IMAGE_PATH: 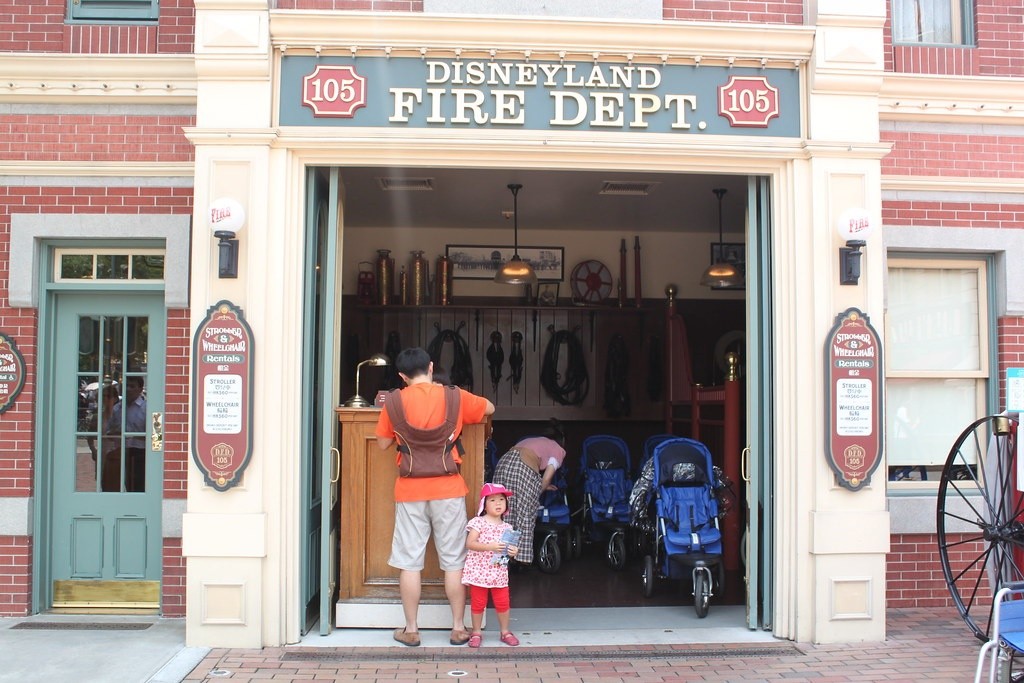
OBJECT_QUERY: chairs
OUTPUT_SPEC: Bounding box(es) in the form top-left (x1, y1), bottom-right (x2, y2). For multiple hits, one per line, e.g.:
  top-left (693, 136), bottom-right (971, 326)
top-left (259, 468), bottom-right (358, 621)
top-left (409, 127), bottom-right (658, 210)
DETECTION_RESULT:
top-left (974, 580), bottom-right (1024, 683)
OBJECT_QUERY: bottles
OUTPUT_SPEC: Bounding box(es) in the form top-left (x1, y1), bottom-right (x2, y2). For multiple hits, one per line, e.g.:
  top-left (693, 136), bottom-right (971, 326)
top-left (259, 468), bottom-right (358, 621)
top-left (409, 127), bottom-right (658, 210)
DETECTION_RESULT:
top-left (377, 248), bottom-right (452, 306)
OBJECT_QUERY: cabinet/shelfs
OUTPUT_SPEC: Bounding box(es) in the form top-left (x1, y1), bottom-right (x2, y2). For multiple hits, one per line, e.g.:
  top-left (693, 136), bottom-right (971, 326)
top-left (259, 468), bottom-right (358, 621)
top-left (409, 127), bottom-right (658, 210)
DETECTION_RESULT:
top-left (335, 405), bottom-right (492, 631)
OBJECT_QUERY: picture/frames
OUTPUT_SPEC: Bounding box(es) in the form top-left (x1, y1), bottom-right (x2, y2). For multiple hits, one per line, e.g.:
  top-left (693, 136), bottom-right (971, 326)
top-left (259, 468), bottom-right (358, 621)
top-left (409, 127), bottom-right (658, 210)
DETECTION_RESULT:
top-left (537, 283), bottom-right (559, 305)
top-left (710, 243), bottom-right (745, 290)
top-left (445, 244), bottom-right (564, 282)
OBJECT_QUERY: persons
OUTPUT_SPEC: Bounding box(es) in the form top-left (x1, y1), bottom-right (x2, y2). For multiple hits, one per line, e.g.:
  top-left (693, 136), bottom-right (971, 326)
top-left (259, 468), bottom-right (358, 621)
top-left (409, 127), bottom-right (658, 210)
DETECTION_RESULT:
top-left (459, 483), bottom-right (520, 648)
top-left (373, 348), bottom-right (496, 647)
top-left (491, 416), bottom-right (569, 575)
top-left (87, 373), bottom-right (147, 493)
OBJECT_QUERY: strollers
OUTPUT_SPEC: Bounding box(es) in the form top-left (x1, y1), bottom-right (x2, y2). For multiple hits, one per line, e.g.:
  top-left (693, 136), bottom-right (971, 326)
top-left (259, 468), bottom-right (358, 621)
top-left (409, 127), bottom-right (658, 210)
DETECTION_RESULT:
top-left (631, 438), bottom-right (741, 619)
top-left (485, 433), bottom-right (685, 573)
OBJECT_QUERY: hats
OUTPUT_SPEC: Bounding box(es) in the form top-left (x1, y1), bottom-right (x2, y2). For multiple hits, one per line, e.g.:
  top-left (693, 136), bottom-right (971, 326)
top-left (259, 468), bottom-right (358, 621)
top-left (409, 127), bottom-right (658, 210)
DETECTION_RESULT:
top-left (477, 483), bottom-right (513, 516)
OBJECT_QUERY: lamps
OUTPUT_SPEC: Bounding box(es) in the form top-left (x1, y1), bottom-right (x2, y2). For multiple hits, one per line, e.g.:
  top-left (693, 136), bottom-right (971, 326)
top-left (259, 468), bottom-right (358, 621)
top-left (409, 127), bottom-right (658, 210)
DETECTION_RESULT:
top-left (207, 199), bottom-right (245, 278)
top-left (494, 185), bottom-right (539, 283)
top-left (345, 353), bottom-right (392, 406)
top-left (699, 188), bottom-right (745, 287)
top-left (838, 204), bottom-right (876, 284)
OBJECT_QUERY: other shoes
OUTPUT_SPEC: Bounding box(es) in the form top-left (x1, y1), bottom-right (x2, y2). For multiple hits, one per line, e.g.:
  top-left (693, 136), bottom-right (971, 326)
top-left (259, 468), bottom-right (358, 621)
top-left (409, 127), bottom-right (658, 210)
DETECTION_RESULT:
top-left (394, 626), bottom-right (420, 646)
top-left (468, 633), bottom-right (481, 647)
top-left (500, 632), bottom-right (518, 646)
top-left (450, 626), bottom-right (473, 645)
